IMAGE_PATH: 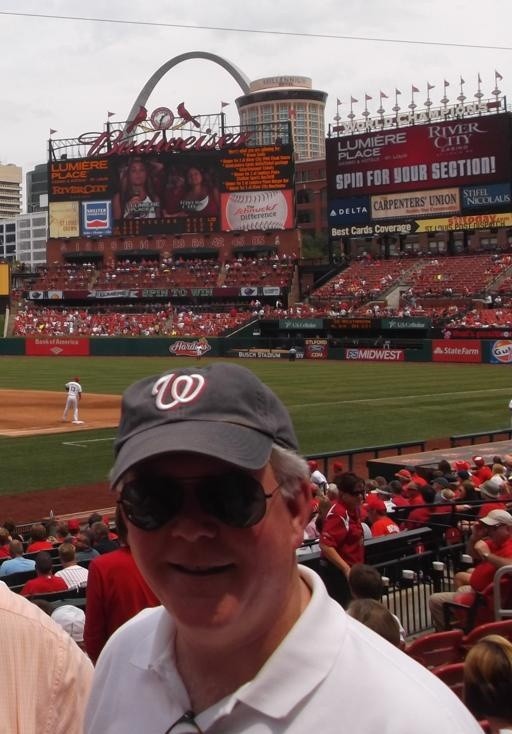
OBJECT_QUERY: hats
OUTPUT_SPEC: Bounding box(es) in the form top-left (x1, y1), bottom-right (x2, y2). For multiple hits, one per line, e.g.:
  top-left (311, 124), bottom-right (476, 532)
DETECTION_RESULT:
top-left (451, 461), bottom-right (472, 471)
top-left (441, 489), bottom-right (456, 501)
top-left (50, 604), bottom-right (86, 642)
top-left (333, 463), bottom-right (342, 472)
top-left (109, 361), bottom-right (300, 494)
top-left (478, 482), bottom-right (500, 498)
top-left (489, 474), bottom-right (508, 489)
top-left (395, 469), bottom-right (411, 480)
top-left (309, 460), bottom-right (317, 470)
top-left (68, 518), bottom-right (79, 530)
top-left (472, 456), bottom-right (485, 467)
top-left (430, 477), bottom-right (448, 488)
top-left (479, 510), bottom-right (512, 526)
top-left (363, 495), bottom-right (385, 510)
top-left (406, 481), bottom-right (419, 490)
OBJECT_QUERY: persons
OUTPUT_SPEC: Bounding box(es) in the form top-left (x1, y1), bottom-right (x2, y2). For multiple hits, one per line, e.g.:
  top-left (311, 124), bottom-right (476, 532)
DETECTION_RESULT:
top-left (196, 345), bottom-right (202, 361)
top-left (113, 156), bottom-right (222, 217)
top-left (289, 347), bottom-right (296, 361)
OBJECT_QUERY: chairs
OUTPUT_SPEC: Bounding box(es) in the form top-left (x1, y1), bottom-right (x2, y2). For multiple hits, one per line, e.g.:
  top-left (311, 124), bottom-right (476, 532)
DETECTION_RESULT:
top-left (1, 524), bottom-right (128, 615)
top-left (398, 620), bottom-right (512, 734)
top-left (443, 578), bottom-right (512, 634)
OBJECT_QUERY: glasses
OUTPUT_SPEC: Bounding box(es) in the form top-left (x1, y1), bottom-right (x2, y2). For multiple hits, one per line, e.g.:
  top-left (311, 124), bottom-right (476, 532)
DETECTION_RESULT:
top-left (114, 471), bottom-right (293, 531)
top-left (166, 711), bottom-right (203, 734)
